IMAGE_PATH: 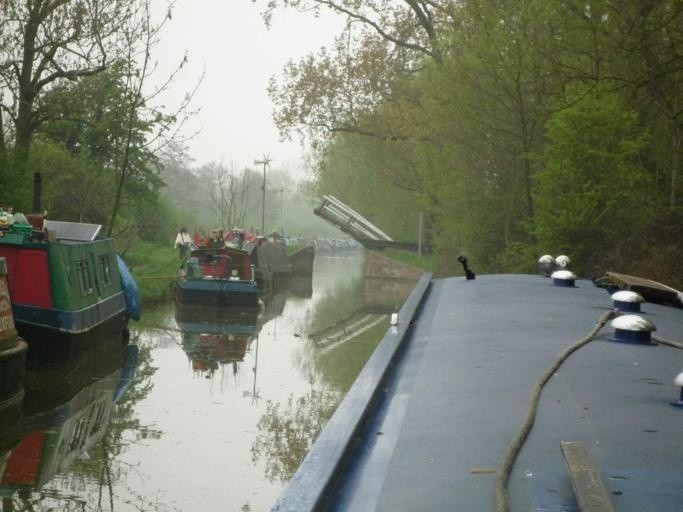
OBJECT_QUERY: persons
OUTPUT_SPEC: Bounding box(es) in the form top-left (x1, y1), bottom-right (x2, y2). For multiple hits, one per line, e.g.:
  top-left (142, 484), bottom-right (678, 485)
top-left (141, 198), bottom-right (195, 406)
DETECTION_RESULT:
top-left (172, 225), bottom-right (193, 269)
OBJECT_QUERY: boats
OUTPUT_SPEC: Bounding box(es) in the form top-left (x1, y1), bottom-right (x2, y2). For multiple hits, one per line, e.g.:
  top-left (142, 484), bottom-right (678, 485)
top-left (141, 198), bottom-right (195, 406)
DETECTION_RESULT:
top-left (0, 172), bottom-right (315, 407)
